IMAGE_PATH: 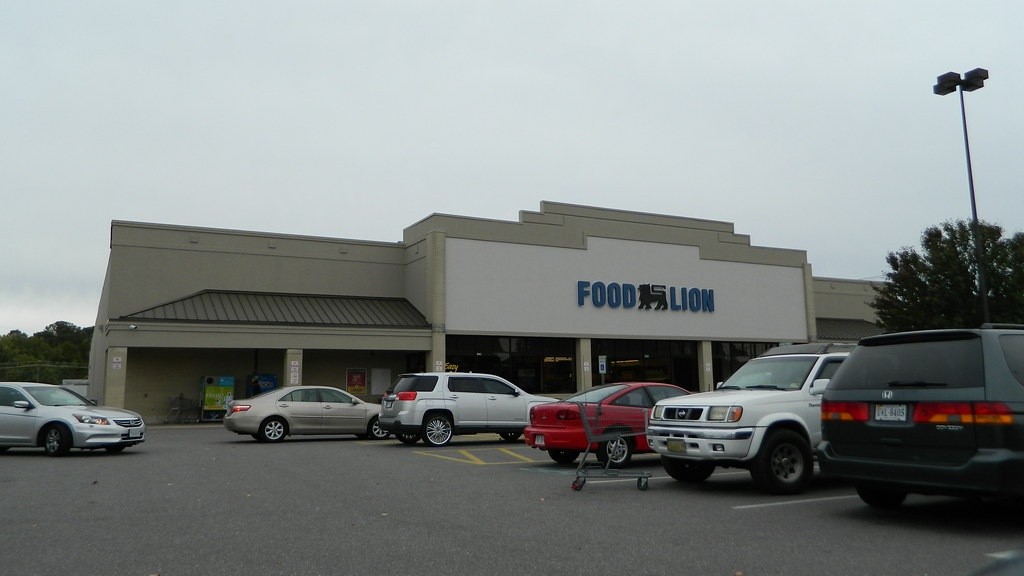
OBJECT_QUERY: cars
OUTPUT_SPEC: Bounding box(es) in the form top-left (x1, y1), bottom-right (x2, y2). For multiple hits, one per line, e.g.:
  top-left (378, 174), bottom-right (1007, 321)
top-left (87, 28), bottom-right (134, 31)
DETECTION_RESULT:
top-left (523, 379), bottom-right (696, 471)
top-left (0, 379), bottom-right (146, 459)
top-left (222, 383), bottom-right (391, 444)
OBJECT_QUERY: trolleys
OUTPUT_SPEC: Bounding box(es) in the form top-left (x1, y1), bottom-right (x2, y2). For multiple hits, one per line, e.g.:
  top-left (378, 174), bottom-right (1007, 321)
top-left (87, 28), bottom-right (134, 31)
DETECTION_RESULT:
top-left (563, 397), bottom-right (655, 492)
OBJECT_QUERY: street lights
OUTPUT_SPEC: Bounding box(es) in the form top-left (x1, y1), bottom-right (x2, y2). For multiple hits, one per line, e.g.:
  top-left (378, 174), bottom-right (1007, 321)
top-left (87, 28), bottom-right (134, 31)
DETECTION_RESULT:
top-left (932, 66), bottom-right (992, 323)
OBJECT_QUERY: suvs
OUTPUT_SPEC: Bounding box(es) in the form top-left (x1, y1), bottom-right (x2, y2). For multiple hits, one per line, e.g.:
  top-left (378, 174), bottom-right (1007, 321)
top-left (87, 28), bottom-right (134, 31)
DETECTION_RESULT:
top-left (645, 341), bottom-right (857, 496)
top-left (378, 370), bottom-right (560, 448)
top-left (817, 321), bottom-right (1024, 511)
